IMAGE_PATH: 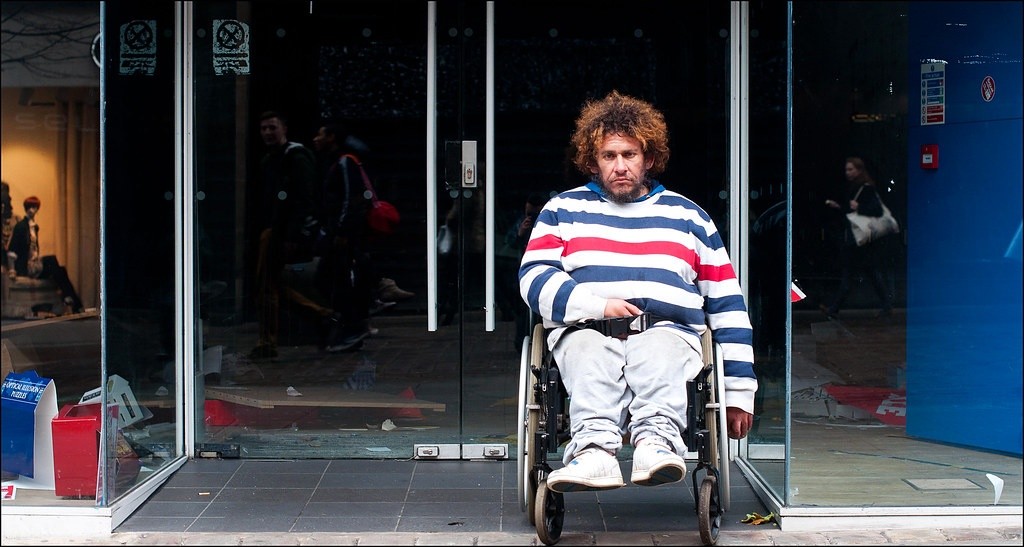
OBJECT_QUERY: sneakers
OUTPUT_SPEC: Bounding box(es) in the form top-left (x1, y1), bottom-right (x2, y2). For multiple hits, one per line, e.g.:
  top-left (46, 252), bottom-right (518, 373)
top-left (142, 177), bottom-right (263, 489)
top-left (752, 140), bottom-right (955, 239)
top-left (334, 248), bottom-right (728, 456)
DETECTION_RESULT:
top-left (546, 447), bottom-right (623, 492)
top-left (631, 436), bottom-right (687, 487)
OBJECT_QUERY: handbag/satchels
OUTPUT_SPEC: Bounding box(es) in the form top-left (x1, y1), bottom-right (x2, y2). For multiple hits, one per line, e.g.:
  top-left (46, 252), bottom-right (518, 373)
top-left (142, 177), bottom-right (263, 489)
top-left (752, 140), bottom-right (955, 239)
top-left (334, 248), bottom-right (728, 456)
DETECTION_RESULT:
top-left (340, 153), bottom-right (400, 234)
top-left (845, 183), bottom-right (900, 247)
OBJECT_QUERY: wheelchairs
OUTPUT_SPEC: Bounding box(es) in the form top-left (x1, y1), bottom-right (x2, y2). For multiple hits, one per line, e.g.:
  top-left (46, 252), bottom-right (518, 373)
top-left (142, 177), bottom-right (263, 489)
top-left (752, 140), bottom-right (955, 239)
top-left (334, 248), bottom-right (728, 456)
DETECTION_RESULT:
top-left (515, 321), bottom-right (733, 546)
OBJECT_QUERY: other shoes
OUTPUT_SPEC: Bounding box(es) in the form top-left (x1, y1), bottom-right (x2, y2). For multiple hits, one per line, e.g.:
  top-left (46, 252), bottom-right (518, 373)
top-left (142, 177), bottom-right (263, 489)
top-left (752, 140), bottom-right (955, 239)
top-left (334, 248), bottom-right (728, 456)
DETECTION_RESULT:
top-left (821, 304), bottom-right (839, 319)
top-left (365, 293), bottom-right (398, 316)
top-left (326, 328), bottom-right (369, 351)
top-left (238, 347), bottom-right (279, 363)
top-left (380, 278), bottom-right (414, 300)
top-left (876, 308), bottom-right (894, 321)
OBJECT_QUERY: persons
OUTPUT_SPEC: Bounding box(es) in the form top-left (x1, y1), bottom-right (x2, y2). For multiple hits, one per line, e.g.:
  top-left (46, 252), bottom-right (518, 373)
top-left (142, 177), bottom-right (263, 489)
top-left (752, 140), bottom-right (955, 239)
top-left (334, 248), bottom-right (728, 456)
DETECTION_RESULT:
top-left (312, 125), bottom-right (414, 351)
top-left (506, 194), bottom-right (545, 353)
top-left (820, 157), bottom-right (896, 319)
top-left (517, 91), bottom-right (758, 492)
top-left (444, 158), bottom-right (485, 310)
top-left (249, 111), bottom-right (344, 359)
top-left (7, 195), bottom-right (96, 315)
